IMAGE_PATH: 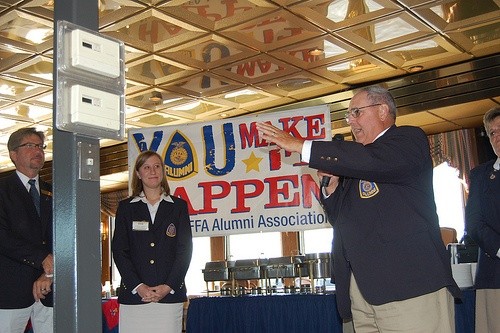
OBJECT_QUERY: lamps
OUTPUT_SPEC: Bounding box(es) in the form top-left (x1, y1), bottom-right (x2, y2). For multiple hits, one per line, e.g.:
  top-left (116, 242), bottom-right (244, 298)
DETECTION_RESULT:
top-left (149, 92), bottom-right (162, 102)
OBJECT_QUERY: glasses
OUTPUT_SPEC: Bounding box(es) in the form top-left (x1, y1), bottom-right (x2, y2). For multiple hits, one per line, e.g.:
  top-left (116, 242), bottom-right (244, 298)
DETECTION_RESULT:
top-left (344, 104), bottom-right (382, 123)
top-left (12, 142), bottom-right (47, 149)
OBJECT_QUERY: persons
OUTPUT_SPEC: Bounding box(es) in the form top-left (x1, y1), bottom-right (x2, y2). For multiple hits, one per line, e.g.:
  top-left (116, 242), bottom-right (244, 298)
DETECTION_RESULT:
top-left (112, 152), bottom-right (192, 333)
top-left (0, 127), bottom-right (54, 333)
top-left (254, 87), bottom-right (464, 333)
top-left (465, 106), bottom-right (500, 333)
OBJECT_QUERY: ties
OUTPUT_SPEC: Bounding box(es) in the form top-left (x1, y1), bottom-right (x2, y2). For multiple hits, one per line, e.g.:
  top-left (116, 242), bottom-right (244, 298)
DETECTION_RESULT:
top-left (28, 179), bottom-right (41, 218)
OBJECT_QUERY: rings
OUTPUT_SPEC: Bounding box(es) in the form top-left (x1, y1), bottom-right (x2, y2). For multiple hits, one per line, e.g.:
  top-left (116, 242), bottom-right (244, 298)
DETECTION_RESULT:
top-left (143, 298), bottom-right (144, 299)
top-left (40, 288), bottom-right (46, 291)
top-left (272, 132), bottom-right (275, 137)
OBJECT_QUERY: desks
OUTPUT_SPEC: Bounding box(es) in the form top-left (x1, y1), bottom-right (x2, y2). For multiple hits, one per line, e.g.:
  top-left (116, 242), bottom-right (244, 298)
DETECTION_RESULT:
top-left (186, 287), bottom-right (478, 332)
